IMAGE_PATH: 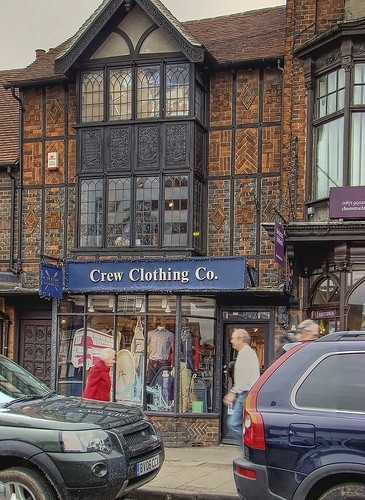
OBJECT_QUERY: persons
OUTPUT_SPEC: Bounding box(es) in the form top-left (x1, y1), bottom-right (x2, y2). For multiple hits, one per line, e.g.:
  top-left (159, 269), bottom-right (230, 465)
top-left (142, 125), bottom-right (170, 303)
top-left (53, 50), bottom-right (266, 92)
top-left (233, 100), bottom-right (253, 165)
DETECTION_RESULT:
top-left (223, 328), bottom-right (261, 452)
top-left (268, 319), bottom-right (320, 371)
top-left (82, 348), bottom-right (117, 402)
top-left (147, 325), bottom-right (175, 388)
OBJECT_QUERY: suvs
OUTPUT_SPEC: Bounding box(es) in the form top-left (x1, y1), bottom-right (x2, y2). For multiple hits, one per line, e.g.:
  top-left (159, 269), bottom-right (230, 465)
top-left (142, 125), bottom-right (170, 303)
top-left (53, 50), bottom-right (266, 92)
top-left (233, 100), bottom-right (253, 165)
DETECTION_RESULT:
top-left (232, 331), bottom-right (365, 500)
top-left (0, 352), bottom-right (166, 499)
